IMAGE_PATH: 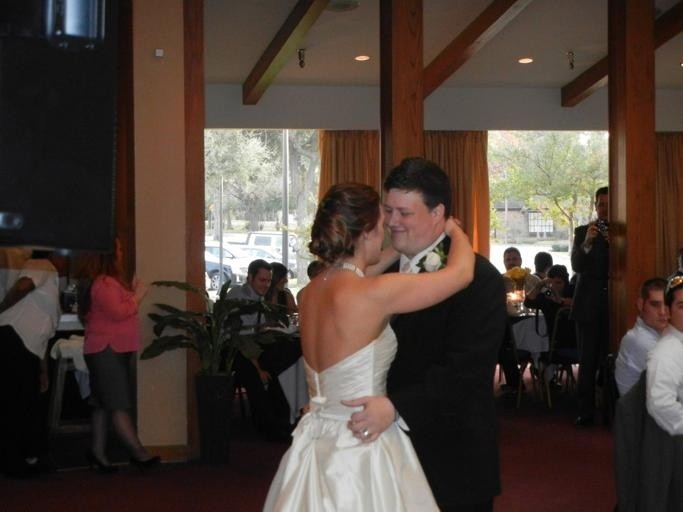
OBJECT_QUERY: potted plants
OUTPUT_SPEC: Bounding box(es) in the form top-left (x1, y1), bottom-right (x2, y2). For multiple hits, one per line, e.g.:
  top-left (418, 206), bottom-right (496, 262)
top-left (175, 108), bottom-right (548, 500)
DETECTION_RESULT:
top-left (140, 269), bottom-right (300, 466)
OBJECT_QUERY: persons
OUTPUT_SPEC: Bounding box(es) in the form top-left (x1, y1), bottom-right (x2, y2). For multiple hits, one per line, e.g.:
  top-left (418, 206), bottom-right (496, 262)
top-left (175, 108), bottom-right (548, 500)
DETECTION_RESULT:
top-left (77, 234), bottom-right (162, 473)
top-left (300, 157), bottom-right (508, 510)
top-left (263, 183), bottom-right (476, 512)
top-left (614, 278), bottom-right (669, 395)
top-left (645, 270), bottom-right (683, 438)
top-left (219, 262), bottom-right (321, 444)
top-left (1, 251), bottom-right (64, 479)
top-left (500, 184), bottom-right (610, 429)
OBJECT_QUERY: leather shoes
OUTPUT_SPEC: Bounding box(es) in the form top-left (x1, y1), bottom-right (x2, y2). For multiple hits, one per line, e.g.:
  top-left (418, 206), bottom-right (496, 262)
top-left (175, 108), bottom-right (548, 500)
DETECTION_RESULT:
top-left (552, 379), bottom-right (563, 390)
top-left (501, 384), bottom-right (526, 392)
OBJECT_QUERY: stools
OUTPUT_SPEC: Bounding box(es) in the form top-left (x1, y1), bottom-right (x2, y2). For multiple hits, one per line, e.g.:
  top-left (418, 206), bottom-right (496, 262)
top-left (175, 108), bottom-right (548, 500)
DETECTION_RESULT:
top-left (46, 339), bottom-right (117, 448)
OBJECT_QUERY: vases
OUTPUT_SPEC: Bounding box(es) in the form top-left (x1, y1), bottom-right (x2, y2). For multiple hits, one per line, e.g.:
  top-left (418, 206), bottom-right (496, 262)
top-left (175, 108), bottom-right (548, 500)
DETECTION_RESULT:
top-left (514, 290), bottom-right (526, 310)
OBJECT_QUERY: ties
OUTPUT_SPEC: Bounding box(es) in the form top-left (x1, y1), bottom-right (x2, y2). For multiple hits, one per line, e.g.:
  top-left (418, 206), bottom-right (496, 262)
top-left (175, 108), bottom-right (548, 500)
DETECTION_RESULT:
top-left (401, 262), bottom-right (412, 273)
top-left (257, 296), bottom-right (262, 325)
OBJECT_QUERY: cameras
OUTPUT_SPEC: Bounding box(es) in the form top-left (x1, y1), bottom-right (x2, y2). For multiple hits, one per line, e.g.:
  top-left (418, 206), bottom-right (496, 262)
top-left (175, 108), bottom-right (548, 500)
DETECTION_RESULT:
top-left (596, 218), bottom-right (609, 239)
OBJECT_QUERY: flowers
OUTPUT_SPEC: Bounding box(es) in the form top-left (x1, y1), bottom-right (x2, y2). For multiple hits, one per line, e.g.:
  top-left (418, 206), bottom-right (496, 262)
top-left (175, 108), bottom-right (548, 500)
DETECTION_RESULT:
top-left (502, 266), bottom-right (530, 304)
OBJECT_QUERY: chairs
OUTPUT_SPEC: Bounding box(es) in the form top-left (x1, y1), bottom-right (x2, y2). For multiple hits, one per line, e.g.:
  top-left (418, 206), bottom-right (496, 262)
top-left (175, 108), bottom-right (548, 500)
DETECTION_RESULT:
top-left (499, 306), bottom-right (578, 408)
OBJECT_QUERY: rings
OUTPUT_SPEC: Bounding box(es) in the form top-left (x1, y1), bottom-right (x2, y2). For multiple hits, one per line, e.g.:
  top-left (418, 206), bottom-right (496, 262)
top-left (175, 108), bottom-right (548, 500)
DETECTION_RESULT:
top-left (362, 429), bottom-right (369, 437)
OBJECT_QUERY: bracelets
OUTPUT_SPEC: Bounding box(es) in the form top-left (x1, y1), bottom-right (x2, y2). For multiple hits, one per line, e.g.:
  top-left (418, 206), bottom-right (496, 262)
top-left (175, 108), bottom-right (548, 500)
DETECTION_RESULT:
top-left (393, 405), bottom-right (402, 421)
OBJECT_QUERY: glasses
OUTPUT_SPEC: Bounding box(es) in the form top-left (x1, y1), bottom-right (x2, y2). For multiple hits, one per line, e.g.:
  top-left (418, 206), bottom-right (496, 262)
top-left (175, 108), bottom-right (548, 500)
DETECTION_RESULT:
top-left (666, 276), bottom-right (683, 294)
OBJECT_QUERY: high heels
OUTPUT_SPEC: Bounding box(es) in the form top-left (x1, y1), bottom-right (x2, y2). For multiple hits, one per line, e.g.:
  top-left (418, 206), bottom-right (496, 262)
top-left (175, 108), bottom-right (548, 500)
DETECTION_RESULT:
top-left (89, 450), bottom-right (160, 472)
